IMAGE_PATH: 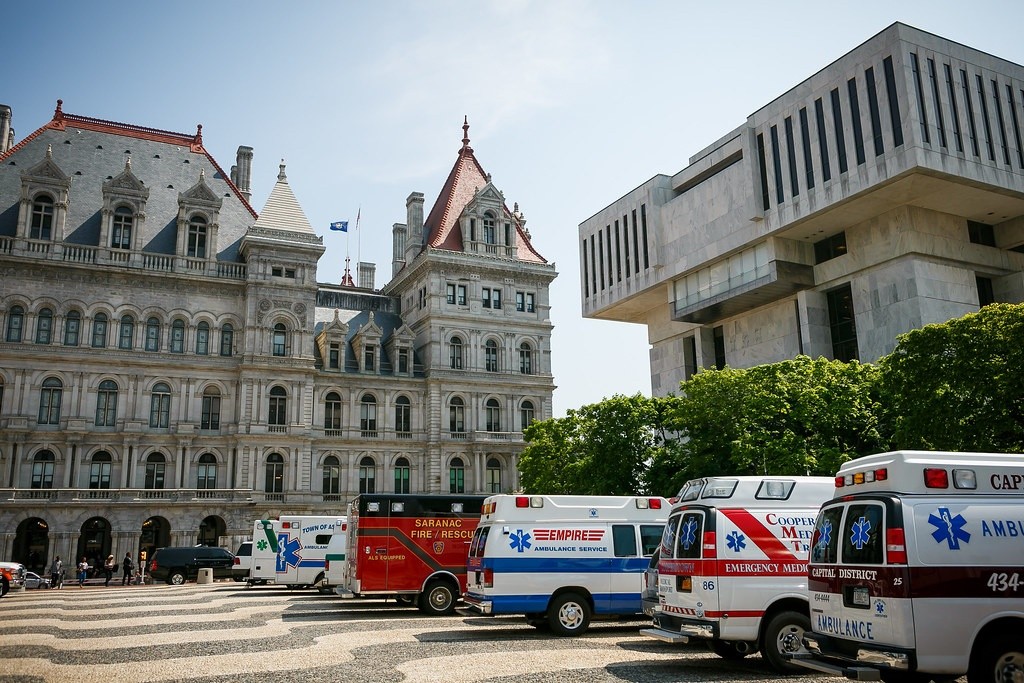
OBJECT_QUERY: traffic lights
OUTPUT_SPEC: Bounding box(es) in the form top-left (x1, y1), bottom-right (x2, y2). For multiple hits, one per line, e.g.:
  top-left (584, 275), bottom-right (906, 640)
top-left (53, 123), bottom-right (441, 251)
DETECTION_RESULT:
top-left (140, 550), bottom-right (147, 561)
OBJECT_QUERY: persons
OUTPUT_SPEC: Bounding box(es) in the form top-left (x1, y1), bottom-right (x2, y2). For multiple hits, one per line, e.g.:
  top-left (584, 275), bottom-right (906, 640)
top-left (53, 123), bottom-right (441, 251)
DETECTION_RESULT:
top-left (47, 547), bottom-right (136, 589)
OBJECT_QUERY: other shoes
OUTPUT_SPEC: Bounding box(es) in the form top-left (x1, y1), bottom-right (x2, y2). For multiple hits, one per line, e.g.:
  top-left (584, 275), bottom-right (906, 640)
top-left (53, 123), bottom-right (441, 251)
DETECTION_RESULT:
top-left (122, 584), bottom-right (126, 586)
top-left (129, 583), bottom-right (133, 586)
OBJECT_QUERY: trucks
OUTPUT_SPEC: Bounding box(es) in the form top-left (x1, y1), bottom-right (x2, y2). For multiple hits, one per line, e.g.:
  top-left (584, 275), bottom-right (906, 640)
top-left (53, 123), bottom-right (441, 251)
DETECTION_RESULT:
top-left (342, 492), bottom-right (501, 616)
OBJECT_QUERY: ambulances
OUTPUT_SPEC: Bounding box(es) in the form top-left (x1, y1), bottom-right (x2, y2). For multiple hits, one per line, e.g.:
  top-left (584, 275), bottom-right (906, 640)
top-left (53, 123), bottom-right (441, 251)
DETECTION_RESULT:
top-left (785, 451), bottom-right (1024, 683)
top-left (462, 491), bottom-right (675, 637)
top-left (249, 515), bottom-right (348, 594)
top-left (639, 475), bottom-right (836, 674)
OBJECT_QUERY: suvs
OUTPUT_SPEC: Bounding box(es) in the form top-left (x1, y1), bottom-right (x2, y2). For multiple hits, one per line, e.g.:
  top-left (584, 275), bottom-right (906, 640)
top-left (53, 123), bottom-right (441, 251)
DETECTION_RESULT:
top-left (0, 561), bottom-right (27, 598)
top-left (149, 546), bottom-right (244, 585)
top-left (641, 539), bottom-right (664, 616)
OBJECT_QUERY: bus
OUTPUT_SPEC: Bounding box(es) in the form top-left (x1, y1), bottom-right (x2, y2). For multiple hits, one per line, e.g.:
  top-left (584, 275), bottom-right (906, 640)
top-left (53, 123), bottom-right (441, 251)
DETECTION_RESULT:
top-left (230, 541), bottom-right (253, 582)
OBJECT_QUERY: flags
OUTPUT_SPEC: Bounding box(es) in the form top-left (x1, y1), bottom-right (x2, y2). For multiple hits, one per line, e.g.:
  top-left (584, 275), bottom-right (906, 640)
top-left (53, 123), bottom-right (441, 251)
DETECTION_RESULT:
top-left (355, 208), bottom-right (361, 229)
top-left (330, 221), bottom-right (348, 232)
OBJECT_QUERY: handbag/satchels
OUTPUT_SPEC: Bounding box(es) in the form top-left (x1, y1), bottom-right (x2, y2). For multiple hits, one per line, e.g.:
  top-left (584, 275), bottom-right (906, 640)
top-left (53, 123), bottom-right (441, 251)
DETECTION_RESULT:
top-left (76, 567), bottom-right (82, 574)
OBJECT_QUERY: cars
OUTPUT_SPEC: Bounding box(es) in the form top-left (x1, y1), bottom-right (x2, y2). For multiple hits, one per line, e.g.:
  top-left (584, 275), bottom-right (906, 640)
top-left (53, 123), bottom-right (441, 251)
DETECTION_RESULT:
top-left (25, 571), bottom-right (51, 589)
